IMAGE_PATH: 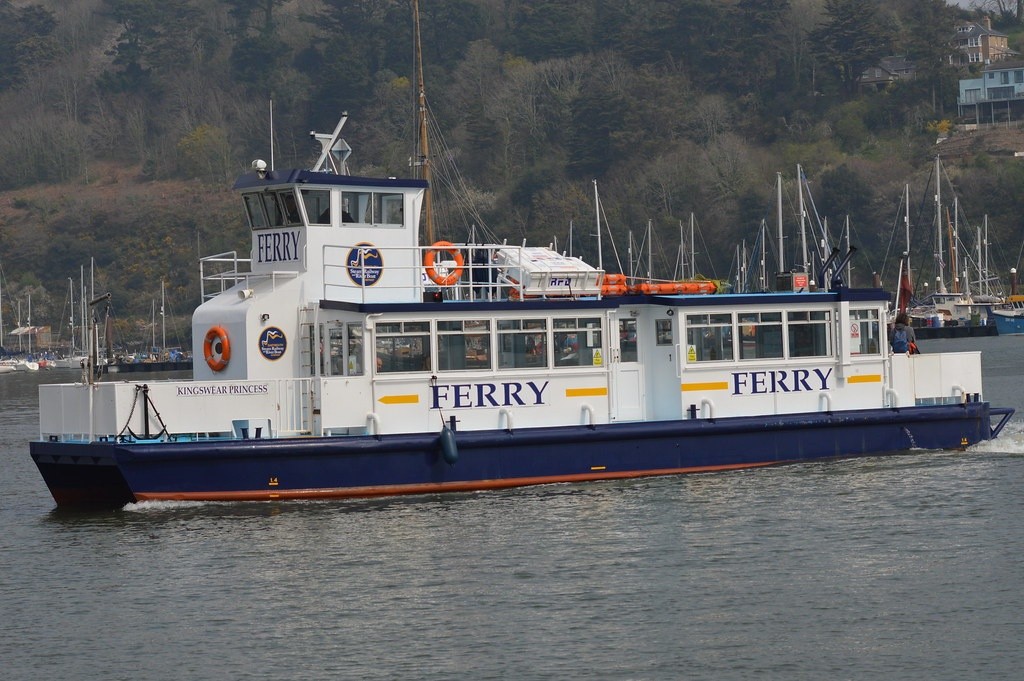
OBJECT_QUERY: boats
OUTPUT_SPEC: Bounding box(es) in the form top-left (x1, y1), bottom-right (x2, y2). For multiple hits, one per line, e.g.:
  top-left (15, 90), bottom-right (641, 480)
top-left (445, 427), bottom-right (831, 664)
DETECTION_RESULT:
top-left (0, 1), bottom-right (1024, 521)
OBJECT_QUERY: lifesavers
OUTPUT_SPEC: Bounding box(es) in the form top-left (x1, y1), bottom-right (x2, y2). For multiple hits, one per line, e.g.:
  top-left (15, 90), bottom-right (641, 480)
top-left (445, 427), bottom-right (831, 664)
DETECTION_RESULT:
top-left (203, 324), bottom-right (230, 371)
top-left (424, 240), bottom-right (463, 285)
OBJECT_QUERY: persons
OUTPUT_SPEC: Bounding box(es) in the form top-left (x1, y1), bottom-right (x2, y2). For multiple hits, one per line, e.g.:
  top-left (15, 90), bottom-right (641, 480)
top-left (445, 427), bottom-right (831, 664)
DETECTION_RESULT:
top-left (276, 196), bottom-right (300, 225)
top-left (890, 314), bottom-right (915, 354)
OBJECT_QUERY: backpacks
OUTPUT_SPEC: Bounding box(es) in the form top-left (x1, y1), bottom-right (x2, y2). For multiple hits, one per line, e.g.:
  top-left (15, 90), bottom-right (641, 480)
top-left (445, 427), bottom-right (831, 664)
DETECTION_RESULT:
top-left (892, 326), bottom-right (908, 353)
